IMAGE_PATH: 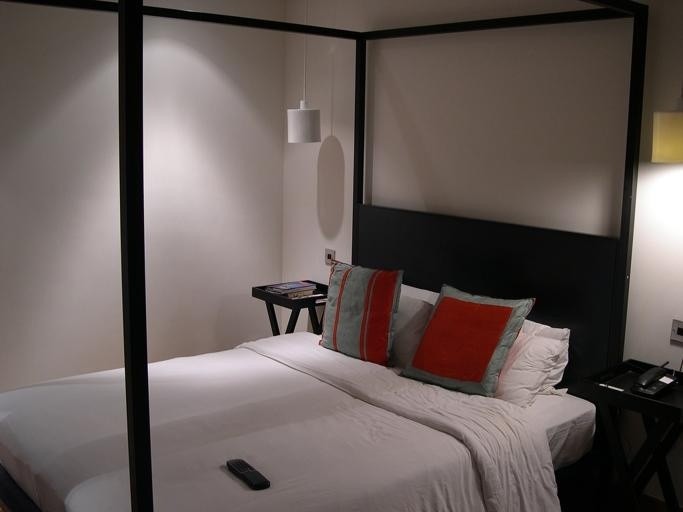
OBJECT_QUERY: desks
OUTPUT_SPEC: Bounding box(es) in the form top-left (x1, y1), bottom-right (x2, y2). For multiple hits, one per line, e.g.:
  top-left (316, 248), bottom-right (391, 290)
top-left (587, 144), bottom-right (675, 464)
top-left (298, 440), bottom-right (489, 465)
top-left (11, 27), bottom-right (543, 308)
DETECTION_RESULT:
top-left (596, 357), bottom-right (683, 512)
top-left (251, 279), bottom-right (329, 335)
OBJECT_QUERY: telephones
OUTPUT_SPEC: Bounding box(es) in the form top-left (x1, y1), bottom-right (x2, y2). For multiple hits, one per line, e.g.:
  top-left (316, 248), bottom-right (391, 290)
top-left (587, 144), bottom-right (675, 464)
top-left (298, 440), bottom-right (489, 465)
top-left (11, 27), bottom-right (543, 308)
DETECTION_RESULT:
top-left (631, 361), bottom-right (678, 398)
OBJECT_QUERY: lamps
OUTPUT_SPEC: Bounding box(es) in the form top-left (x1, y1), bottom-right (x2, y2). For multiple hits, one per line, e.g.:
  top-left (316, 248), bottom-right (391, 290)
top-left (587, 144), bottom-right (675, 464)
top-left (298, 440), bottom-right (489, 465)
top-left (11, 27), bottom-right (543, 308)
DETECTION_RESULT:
top-left (287, 0), bottom-right (321, 144)
top-left (649, 111), bottom-right (683, 165)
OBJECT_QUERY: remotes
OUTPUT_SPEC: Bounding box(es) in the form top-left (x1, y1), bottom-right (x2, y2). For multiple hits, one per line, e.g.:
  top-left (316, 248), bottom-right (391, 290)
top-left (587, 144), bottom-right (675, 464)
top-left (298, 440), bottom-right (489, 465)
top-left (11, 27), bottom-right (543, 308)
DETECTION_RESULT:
top-left (226, 458), bottom-right (270, 490)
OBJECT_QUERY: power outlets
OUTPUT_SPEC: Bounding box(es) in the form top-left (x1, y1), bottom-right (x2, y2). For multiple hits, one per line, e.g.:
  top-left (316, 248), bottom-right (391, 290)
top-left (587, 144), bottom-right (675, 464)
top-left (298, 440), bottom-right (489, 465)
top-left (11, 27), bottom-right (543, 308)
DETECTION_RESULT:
top-left (670, 320), bottom-right (683, 346)
top-left (324, 248), bottom-right (335, 265)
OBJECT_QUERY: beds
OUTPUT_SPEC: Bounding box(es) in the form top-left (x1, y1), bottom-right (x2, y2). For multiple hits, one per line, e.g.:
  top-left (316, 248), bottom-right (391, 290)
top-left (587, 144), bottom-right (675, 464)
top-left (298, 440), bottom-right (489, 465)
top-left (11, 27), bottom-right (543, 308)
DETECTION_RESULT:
top-left (0, 0), bottom-right (649, 512)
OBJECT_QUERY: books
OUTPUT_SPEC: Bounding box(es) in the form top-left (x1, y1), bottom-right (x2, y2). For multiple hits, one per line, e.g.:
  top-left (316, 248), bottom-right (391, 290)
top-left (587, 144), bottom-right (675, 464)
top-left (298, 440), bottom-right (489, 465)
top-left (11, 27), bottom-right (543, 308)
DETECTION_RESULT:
top-left (279, 289), bottom-right (315, 298)
top-left (264, 280), bottom-right (315, 293)
top-left (290, 292), bottom-right (324, 301)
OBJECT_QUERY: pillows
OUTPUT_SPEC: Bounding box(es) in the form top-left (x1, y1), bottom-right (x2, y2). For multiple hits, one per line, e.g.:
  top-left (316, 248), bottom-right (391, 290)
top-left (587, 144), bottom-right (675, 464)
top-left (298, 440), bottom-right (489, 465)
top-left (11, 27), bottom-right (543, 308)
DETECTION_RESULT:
top-left (320, 258), bottom-right (570, 407)
